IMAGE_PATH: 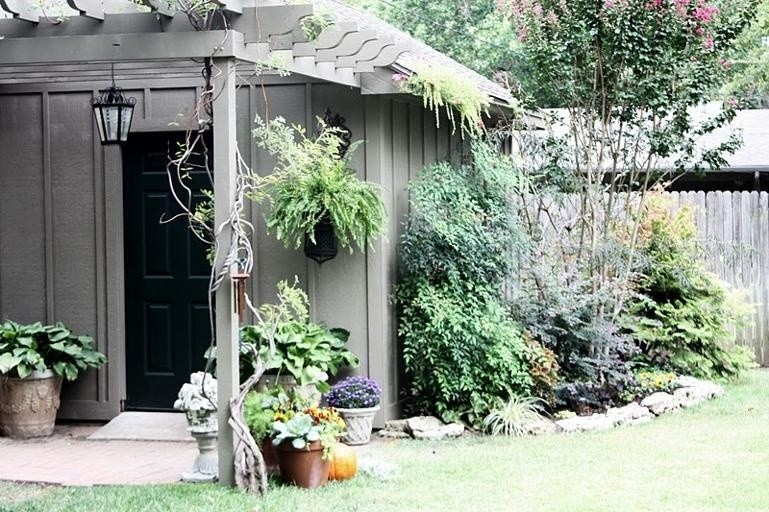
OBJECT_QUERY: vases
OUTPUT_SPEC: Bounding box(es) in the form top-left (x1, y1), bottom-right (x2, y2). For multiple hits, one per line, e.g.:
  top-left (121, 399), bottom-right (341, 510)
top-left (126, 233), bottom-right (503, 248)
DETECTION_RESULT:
top-left (332, 400), bottom-right (379, 447)
top-left (280, 442), bottom-right (329, 490)
top-left (189, 419), bottom-right (218, 476)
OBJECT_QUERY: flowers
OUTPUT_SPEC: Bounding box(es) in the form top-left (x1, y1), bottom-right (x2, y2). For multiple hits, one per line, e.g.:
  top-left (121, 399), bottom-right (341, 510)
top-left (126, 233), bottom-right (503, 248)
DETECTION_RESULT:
top-left (264, 402), bottom-right (341, 462)
top-left (169, 371), bottom-right (216, 420)
top-left (322, 373), bottom-right (379, 404)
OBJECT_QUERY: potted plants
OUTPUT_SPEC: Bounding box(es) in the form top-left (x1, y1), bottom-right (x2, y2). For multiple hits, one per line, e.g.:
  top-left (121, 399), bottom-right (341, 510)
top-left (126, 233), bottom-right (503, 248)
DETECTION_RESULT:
top-left (237, 112), bottom-right (393, 267)
top-left (240, 390), bottom-right (281, 467)
top-left (0, 315), bottom-right (104, 449)
top-left (221, 273), bottom-right (365, 408)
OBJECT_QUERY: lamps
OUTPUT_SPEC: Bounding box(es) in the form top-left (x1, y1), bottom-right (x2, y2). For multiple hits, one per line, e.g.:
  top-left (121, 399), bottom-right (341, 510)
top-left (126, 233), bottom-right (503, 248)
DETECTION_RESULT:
top-left (84, 60), bottom-right (136, 145)
